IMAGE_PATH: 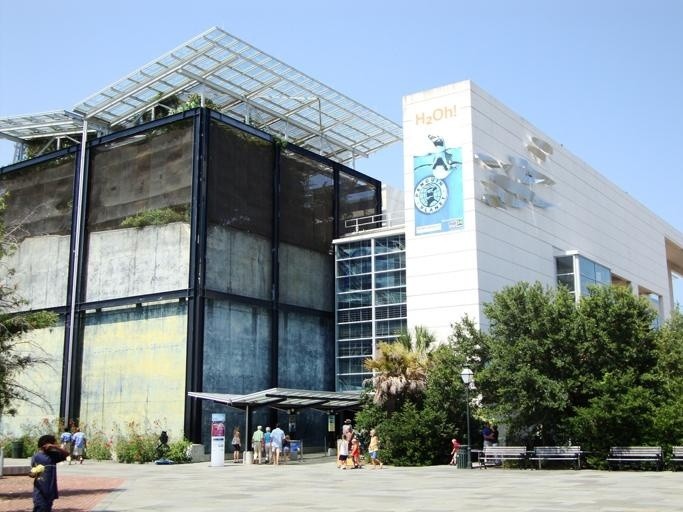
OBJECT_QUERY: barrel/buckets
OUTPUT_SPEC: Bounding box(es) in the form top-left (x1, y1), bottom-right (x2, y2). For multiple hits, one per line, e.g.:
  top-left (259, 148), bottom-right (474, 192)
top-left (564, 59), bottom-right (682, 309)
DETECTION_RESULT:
top-left (242, 452), bottom-right (254, 465)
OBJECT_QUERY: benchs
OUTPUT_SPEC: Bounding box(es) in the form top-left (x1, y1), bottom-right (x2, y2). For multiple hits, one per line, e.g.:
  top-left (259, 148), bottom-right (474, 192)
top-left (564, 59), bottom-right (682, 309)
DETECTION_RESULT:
top-left (670, 446), bottom-right (683, 471)
top-left (606, 446), bottom-right (663, 467)
top-left (478, 446), bottom-right (526, 469)
top-left (528, 445), bottom-right (583, 468)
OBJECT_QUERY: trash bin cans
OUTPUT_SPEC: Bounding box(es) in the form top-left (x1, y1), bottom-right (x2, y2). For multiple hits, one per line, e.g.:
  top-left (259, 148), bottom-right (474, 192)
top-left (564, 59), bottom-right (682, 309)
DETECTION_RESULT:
top-left (12, 441), bottom-right (24, 458)
top-left (456, 445), bottom-right (468, 468)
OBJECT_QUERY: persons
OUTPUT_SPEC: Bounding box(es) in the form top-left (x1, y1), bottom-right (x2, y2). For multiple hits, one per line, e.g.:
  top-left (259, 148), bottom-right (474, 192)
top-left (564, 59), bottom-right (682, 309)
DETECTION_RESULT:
top-left (481, 421), bottom-right (495, 463)
top-left (486, 424), bottom-right (502, 464)
top-left (262, 427), bottom-right (273, 464)
top-left (72, 427), bottom-right (88, 465)
top-left (28, 434), bottom-right (71, 512)
top-left (336, 418), bottom-right (384, 471)
top-left (232, 424), bottom-right (243, 463)
top-left (251, 425), bottom-right (265, 465)
top-left (58, 428), bottom-right (74, 466)
top-left (270, 422), bottom-right (286, 465)
top-left (281, 435), bottom-right (291, 463)
top-left (449, 438), bottom-right (460, 465)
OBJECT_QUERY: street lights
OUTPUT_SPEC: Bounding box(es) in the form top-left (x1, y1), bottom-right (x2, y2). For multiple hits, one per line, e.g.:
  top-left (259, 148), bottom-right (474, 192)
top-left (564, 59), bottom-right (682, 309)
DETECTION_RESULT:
top-left (461, 369), bottom-right (474, 466)
